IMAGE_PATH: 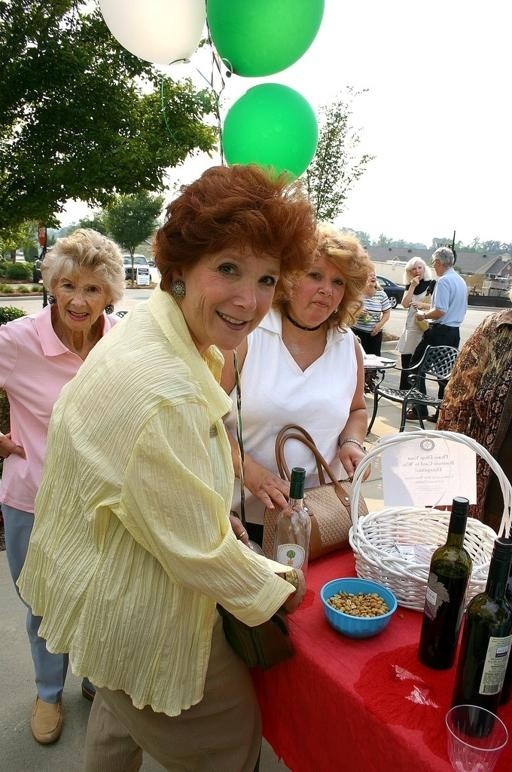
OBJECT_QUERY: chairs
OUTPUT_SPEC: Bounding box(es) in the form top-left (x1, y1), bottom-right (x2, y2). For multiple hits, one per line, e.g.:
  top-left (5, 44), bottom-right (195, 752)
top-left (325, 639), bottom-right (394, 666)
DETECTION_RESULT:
top-left (366, 344), bottom-right (459, 436)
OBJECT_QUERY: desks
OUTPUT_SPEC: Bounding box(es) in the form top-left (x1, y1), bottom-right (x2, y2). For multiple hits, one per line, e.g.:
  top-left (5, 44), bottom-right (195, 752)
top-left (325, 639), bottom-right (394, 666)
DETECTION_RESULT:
top-left (250, 548), bottom-right (512, 772)
top-left (365, 354), bottom-right (396, 393)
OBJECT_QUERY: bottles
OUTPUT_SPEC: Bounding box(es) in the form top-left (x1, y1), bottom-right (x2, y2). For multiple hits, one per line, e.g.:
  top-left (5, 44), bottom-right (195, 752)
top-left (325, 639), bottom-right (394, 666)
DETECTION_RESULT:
top-left (272, 466), bottom-right (312, 583)
top-left (415, 496), bottom-right (473, 672)
top-left (451, 536), bottom-right (512, 739)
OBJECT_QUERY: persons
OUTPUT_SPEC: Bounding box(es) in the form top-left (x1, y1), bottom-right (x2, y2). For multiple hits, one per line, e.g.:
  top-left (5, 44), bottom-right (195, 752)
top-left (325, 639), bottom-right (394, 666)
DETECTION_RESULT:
top-left (434, 282), bottom-right (512, 541)
top-left (346, 262), bottom-right (392, 393)
top-left (0, 226), bottom-right (130, 749)
top-left (403, 246), bottom-right (467, 423)
top-left (397, 256), bottom-right (437, 400)
top-left (219, 219), bottom-right (370, 547)
top-left (12, 162), bottom-right (322, 770)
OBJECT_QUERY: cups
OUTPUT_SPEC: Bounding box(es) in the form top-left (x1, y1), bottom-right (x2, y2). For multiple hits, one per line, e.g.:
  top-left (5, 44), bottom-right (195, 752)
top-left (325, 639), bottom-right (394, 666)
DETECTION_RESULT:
top-left (444, 703), bottom-right (510, 772)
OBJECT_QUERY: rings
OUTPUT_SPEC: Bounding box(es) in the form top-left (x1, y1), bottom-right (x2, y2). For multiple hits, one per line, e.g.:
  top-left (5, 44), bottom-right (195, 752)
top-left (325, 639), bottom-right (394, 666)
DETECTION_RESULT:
top-left (237, 530), bottom-right (246, 540)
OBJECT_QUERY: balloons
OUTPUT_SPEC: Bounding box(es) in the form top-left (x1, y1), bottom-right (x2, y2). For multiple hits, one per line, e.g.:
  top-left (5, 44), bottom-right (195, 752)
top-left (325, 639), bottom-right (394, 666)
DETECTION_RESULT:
top-left (221, 79), bottom-right (318, 188)
top-left (100, 0), bottom-right (205, 69)
top-left (205, 1), bottom-right (325, 79)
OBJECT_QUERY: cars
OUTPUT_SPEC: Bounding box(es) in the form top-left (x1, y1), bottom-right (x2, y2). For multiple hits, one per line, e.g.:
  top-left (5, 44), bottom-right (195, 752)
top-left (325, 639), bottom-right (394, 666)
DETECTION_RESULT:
top-left (374, 276), bottom-right (406, 309)
top-left (34, 246), bottom-right (53, 281)
top-left (17, 252), bottom-right (24, 256)
top-left (148, 260), bottom-right (156, 267)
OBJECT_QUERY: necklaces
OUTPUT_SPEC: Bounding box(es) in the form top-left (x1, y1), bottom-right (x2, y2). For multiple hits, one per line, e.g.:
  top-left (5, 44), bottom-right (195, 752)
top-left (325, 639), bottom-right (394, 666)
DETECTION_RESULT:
top-left (286, 313), bottom-right (323, 332)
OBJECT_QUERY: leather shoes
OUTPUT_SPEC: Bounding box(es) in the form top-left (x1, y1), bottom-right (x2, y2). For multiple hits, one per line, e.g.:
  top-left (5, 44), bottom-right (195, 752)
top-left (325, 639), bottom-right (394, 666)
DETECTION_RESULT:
top-left (30, 693), bottom-right (66, 746)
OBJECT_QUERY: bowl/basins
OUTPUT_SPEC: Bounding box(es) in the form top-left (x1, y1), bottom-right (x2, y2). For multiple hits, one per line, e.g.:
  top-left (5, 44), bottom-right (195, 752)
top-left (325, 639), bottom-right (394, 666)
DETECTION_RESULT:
top-left (318, 577), bottom-right (398, 639)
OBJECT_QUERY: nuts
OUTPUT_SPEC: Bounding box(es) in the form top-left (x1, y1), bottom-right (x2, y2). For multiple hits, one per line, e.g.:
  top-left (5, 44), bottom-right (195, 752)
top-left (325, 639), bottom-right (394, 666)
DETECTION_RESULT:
top-left (327, 589), bottom-right (390, 618)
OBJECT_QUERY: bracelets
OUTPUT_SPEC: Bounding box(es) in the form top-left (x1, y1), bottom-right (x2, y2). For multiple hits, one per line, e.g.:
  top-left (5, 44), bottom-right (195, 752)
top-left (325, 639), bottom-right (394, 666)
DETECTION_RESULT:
top-left (339, 437), bottom-right (366, 453)
top-left (283, 567), bottom-right (300, 604)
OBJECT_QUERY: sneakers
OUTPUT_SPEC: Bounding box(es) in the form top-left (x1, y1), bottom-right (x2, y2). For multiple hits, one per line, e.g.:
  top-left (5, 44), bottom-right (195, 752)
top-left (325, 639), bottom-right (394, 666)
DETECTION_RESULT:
top-left (427, 414), bottom-right (438, 422)
top-left (405, 408), bottom-right (428, 420)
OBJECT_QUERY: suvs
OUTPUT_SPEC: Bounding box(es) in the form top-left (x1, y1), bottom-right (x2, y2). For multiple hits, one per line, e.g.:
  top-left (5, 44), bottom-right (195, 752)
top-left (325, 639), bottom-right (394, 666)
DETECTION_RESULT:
top-left (122, 254), bottom-right (150, 278)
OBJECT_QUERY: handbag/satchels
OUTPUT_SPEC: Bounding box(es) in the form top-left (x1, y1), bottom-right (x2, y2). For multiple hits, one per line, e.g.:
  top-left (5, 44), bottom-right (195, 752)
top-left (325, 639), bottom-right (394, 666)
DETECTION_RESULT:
top-left (262, 423), bottom-right (370, 562)
top-left (217, 598), bottom-right (294, 670)
